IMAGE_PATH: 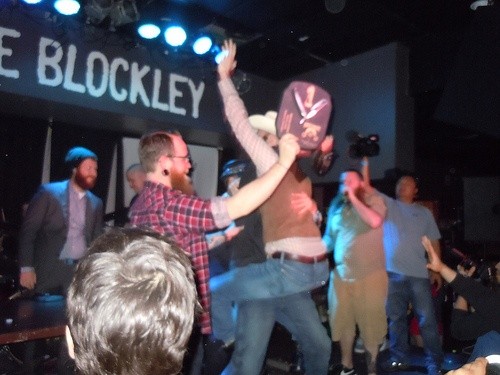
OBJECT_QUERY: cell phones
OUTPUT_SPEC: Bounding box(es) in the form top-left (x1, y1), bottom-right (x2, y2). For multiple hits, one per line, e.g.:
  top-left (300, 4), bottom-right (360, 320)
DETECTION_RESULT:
top-left (485, 363), bottom-right (500, 375)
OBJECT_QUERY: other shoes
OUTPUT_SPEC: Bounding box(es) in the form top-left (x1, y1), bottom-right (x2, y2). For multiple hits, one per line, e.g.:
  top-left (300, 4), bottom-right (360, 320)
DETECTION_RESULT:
top-left (380, 357), bottom-right (408, 372)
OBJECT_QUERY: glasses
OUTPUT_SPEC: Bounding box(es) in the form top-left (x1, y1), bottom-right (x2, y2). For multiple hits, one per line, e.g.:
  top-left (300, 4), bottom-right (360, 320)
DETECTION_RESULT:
top-left (166, 153), bottom-right (191, 160)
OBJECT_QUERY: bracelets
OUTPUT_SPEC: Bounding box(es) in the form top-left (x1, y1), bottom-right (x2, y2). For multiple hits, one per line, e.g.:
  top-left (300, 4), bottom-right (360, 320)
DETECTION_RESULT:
top-left (276, 160), bottom-right (290, 173)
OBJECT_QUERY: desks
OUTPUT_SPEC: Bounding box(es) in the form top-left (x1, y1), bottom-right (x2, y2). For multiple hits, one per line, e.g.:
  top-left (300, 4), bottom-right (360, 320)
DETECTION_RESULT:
top-left (0, 292), bottom-right (69, 375)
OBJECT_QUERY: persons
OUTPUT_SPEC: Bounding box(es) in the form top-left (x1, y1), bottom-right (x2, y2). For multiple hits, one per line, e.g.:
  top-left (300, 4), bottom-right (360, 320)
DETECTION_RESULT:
top-left (357, 132), bottom-right (447, 375)
top-left (219, 109), bottom-right (332, 375)
top-left (125, 163), bottom-right (147, 200)
top-left (207, 39), bottom-right (330, 357)
top-left (321, 168), bottom-right (387, 375)
top-left (201, 157), bottom-right (249, 276)
top-left (405, 221), bottom-right (500, 375)
top-left (129, 131), bottom-right (300, 375)
top-left (61, 224), bottom-right (195, 375)
top-left (16, 145), bottom-right (103, 375)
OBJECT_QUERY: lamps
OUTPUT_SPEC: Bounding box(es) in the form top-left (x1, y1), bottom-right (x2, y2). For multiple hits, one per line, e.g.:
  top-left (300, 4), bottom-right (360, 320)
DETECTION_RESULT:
top-left (22, 0), bottom-right (214, 54)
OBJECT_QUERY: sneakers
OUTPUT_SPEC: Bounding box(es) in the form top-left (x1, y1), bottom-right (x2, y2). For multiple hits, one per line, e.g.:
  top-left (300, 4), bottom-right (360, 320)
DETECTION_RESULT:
top-left (328, 364), bottom-right (355, 375)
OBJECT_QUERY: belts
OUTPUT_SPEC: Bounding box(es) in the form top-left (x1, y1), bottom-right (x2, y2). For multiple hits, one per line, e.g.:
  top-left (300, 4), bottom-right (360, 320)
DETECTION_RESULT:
top-left (272, 250), bottom-right (327, 263)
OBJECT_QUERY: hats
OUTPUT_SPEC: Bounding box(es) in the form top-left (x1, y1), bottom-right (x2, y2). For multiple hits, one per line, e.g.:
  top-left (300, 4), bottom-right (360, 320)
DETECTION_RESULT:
top-left (249, 110), bottom-right (278, 133)
top-left (65, 147), bottom-right (98, 168)
top-left (222, 158), bottom-right (254, 176)
